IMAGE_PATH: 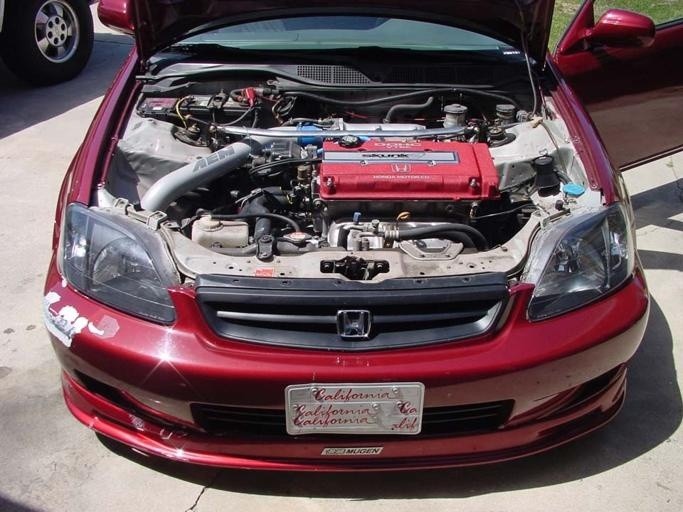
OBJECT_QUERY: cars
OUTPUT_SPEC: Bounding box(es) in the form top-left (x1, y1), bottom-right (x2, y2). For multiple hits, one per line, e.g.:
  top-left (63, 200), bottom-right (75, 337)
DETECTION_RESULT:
top-left (0, 0), bottom-right (100, 82)
top-left (45, 1), bottom-right (682, 475)
